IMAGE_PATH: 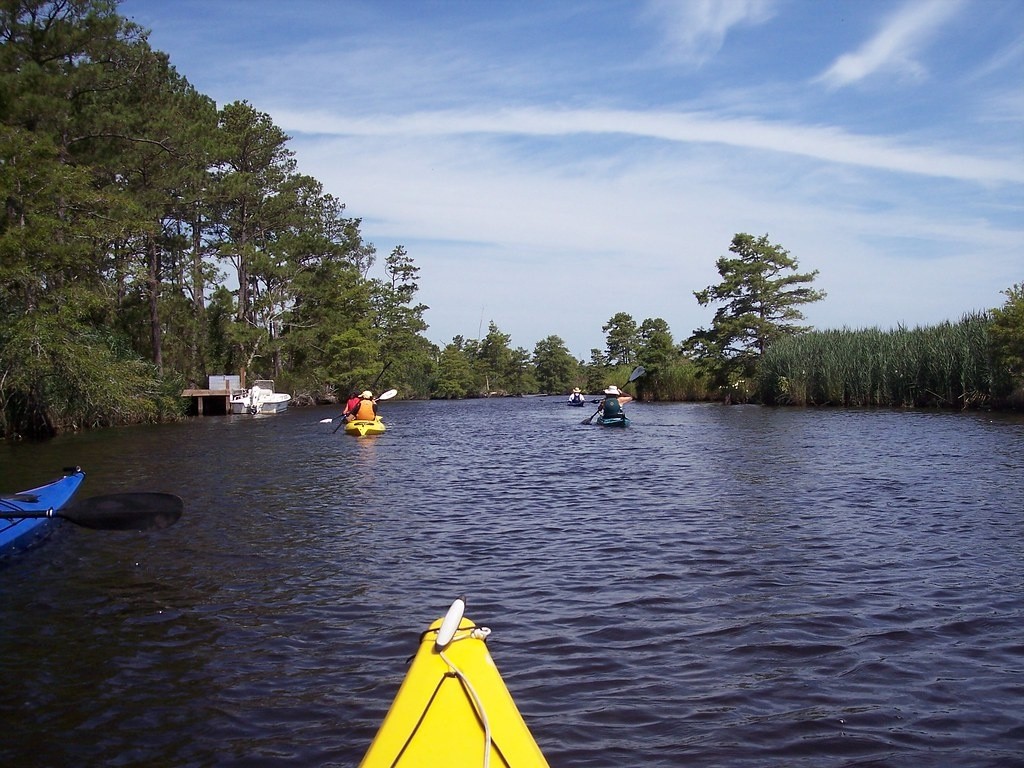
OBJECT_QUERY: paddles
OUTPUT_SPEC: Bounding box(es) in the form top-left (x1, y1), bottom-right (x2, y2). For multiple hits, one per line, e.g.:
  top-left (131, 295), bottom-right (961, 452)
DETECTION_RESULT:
top-left (330, 360), bottom-right (394, 436)
top-left (0, 484), bottom-right (192, 540)
top-left (318, 388), bottom-right (399, 425)
top-left (579, 363), bottom-right (648, 428)
top-left (551, 397), bottom-right (603, 405)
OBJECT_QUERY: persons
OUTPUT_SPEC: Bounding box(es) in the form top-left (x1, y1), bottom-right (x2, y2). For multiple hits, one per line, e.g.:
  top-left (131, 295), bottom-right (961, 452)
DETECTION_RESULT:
top-left (569, 387), bottom-right (585, 403)
top-left (343, 391), bottom-right (378, 424)
top-left (598, 386), bottom-right (633, 416)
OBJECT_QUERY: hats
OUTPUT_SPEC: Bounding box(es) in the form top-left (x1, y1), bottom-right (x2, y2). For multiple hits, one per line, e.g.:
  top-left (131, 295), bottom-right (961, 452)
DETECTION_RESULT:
top-left (573, 388), bottom-right (581, 393)
top-left (604, 385), bottom-right (620, 395)
top-left (358, 390), bottom-right (373, 399)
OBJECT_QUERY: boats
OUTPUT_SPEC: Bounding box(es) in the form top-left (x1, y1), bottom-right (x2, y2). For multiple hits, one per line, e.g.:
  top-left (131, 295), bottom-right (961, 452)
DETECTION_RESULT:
top-left (344, 415), bottom-right (386, 436)
top-left (229, 385), bottom-right (291, 415)
top-left (567, 399), bottom-right (630, 428)
top-left (0, 464), bottom-right (88, 559)
